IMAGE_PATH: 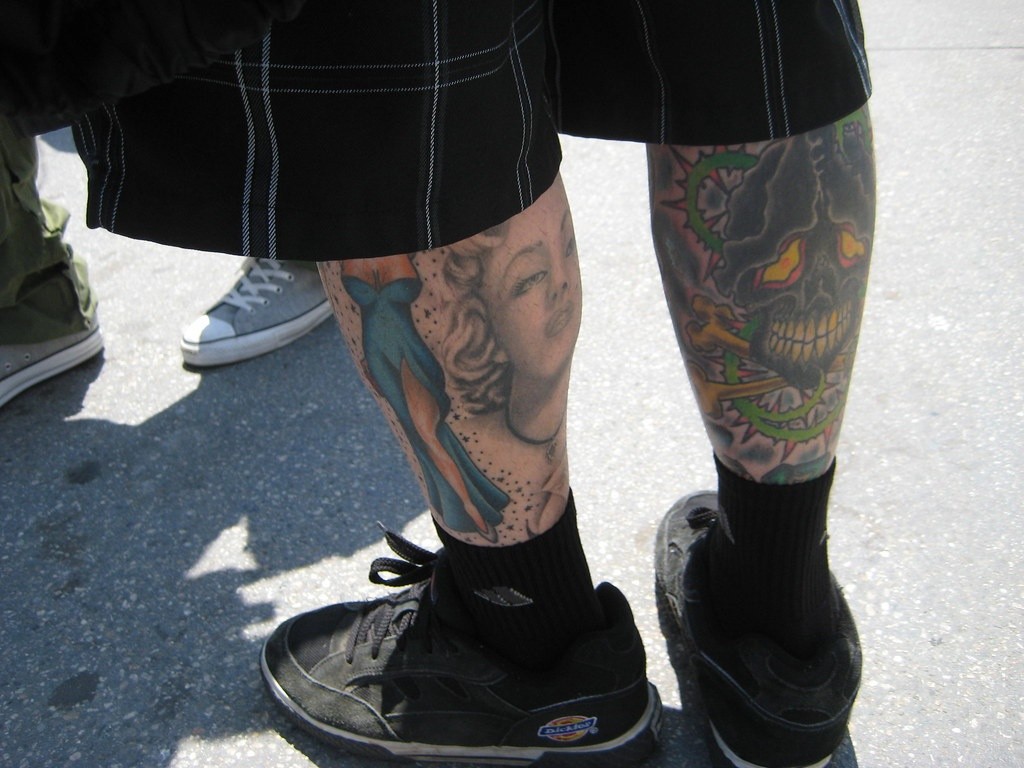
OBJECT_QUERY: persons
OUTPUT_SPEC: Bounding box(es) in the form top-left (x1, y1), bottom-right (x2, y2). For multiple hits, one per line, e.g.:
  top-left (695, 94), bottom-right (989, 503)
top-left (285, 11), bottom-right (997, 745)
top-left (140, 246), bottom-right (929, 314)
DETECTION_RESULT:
top-left (0, 0), bottom-right (877, 768)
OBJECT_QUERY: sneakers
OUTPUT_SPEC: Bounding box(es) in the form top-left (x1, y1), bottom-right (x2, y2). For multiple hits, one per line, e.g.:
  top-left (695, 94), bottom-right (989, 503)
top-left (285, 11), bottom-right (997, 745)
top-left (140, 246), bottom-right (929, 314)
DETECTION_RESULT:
top-left (0, 327), bottom-right (104, 407)
top-left (259, 520), bottom-right (663, 768)
top-left (181, 252), bottom-right (334, 366)
top-left (654, 488), bottom-right (862, 768)
